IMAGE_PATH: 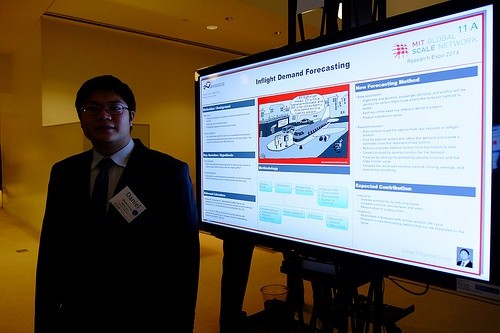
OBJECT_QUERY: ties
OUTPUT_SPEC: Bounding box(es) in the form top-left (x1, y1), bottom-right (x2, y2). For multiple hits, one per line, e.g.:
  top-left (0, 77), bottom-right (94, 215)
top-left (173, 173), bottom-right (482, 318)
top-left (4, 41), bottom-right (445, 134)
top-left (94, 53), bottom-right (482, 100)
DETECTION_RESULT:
top-left (91, 159), bottom-right (114, 216)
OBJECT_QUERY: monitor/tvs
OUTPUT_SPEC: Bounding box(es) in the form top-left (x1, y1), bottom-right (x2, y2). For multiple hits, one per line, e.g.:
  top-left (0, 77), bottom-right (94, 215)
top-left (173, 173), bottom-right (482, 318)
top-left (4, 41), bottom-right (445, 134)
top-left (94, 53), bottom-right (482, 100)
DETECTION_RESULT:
top-left (194, 0), bottom-right (500, 303)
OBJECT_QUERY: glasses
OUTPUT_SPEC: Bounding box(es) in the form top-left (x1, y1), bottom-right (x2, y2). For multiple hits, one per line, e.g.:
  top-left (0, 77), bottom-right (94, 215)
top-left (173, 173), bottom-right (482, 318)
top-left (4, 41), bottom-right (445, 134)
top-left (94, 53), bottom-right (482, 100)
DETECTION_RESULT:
top-left (79, 104), bottom-right (128, 115)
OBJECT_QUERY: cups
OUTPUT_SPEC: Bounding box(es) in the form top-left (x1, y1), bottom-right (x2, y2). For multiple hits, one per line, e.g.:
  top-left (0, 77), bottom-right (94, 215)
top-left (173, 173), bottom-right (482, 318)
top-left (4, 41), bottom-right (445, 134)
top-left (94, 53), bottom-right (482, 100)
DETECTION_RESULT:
top-left (260, 284), bottom-right (288, 320)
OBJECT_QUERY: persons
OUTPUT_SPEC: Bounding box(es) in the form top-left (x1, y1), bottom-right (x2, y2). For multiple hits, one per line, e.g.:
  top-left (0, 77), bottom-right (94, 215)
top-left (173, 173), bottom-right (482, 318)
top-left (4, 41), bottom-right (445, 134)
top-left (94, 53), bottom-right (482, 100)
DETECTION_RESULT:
top-left (457, 249), bottom-right (472, 268)
top-left (34, 75), bottom-right (200, 333)
